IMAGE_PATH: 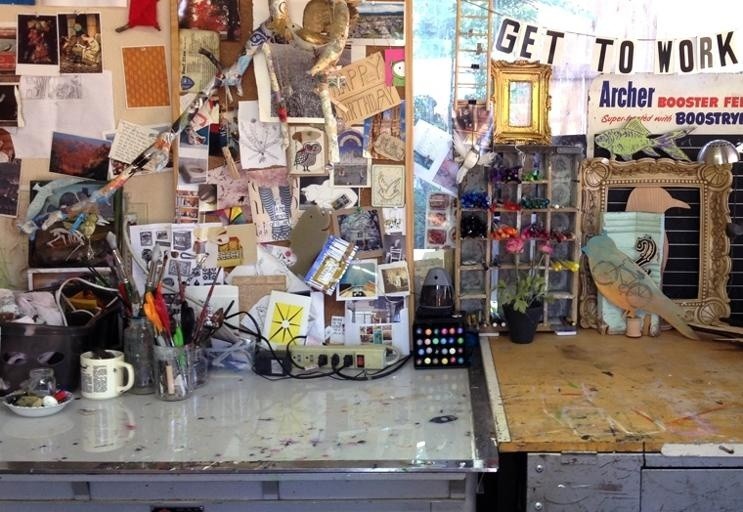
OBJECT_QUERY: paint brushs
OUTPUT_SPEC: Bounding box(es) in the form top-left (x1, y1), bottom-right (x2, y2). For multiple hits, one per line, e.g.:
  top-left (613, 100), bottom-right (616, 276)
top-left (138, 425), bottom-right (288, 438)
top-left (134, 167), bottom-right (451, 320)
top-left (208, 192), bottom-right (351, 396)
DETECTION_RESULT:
top-left (105, 231), bottom-right (235, 344)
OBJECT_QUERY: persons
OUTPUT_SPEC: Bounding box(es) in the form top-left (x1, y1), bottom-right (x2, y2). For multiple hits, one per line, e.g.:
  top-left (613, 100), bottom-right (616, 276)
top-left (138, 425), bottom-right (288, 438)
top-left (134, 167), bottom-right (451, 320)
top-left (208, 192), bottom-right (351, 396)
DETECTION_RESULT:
top-left (23, 19), bottom-right (99, 65)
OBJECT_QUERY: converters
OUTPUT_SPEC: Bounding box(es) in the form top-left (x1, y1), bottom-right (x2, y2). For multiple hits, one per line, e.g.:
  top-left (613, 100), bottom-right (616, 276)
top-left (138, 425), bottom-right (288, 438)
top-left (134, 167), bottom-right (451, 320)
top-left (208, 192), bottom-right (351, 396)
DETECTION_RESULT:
top-left (253, 349), bottom-right (292, 378)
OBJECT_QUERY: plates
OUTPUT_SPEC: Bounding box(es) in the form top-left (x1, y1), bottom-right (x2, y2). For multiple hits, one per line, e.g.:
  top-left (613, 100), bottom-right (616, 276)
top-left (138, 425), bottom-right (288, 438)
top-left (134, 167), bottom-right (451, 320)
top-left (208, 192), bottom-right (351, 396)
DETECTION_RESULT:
top-left (1, 388), bottom-right (73, 418)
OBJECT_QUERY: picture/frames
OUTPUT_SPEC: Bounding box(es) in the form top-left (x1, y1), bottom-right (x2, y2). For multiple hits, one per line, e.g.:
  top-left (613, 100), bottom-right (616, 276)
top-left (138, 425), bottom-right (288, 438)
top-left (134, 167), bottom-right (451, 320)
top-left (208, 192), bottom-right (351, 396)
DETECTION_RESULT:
top-left (490, 58), bottom-right (554, 146)
top-left (580, 157), bottom-right (733, 335)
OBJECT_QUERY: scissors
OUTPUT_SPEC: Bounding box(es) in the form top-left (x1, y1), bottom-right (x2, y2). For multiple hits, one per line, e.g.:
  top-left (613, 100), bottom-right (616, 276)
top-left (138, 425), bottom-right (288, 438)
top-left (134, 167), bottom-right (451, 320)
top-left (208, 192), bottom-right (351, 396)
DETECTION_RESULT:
top-left (142, 286), bottom-right (195, 347)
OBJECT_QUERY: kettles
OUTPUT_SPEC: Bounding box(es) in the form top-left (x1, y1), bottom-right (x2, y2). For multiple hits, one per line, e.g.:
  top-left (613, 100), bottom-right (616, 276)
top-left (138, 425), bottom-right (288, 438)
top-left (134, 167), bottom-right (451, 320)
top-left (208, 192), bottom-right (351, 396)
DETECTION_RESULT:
top-left (416, 267), bottom-right (455, 318)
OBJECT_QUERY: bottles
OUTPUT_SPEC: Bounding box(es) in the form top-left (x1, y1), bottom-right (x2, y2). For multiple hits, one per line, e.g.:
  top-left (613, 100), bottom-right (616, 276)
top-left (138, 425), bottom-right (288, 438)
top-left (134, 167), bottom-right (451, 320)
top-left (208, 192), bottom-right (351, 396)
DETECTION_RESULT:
top-left (123, 317), bottom-right (154, 395)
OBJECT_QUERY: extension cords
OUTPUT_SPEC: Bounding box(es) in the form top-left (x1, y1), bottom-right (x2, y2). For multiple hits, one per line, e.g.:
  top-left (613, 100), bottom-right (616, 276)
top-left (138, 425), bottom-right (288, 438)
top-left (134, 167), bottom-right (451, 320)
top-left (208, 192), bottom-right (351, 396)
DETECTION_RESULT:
top-left (276, 346), bottom-right (387, 370)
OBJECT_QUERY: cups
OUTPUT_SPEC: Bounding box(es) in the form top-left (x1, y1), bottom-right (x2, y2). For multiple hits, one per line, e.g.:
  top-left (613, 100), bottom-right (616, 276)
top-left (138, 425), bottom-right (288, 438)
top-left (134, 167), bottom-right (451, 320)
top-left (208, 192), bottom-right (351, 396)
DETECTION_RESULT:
top-left (79, 349), bottom-right (134, 401)
top-left (153, 344), bottom-right (192, 402)
